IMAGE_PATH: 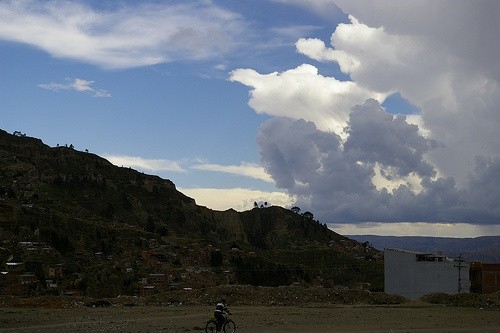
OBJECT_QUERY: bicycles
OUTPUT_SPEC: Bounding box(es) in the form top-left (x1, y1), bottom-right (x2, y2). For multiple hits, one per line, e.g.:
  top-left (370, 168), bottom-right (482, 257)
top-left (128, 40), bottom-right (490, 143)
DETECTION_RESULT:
top-left (205, 312), bottom-right (236, 333)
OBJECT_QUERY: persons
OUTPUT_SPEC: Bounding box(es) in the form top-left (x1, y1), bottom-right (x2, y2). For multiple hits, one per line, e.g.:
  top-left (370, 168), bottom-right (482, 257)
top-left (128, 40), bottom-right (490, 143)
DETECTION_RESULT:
top-left (213, 298), bottom-right (232, 333)
